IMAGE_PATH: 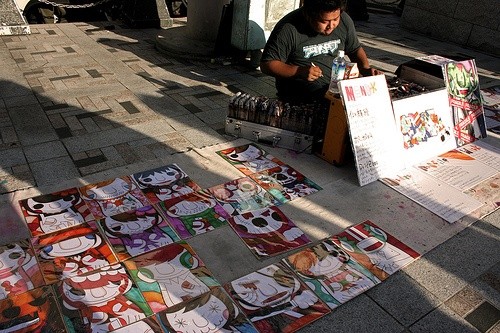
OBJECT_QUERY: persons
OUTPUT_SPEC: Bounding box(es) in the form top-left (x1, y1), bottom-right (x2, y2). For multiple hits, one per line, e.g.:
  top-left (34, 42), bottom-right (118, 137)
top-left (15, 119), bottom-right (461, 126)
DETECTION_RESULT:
top-left (258, 0), bottom-right (384, 166)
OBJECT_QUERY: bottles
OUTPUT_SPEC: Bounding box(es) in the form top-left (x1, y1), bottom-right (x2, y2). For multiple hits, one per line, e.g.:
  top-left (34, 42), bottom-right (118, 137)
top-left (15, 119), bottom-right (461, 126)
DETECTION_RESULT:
top-left (328, 50), bottom-right (347, 94)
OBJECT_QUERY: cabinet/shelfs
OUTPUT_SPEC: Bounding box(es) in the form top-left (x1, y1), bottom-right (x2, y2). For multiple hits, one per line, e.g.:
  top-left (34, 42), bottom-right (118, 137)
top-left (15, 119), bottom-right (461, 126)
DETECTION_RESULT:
top-left (321, 90), bottom-right (354, 167)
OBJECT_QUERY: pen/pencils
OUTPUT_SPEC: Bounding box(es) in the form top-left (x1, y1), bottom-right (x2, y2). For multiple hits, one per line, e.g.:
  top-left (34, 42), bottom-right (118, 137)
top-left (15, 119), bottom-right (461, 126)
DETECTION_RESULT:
top-left (311, 62), bottom-right (324, 77)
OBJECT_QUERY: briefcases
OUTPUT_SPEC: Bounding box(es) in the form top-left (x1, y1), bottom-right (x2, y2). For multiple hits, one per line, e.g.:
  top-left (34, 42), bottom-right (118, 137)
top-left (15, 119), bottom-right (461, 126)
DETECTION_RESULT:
top-left (225, 117), bottom-right (315, 154)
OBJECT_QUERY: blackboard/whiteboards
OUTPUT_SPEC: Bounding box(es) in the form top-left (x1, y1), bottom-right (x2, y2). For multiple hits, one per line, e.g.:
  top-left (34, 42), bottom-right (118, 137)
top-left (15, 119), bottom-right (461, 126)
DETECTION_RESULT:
top-left (339, 73), bottom-right (409, 185)
